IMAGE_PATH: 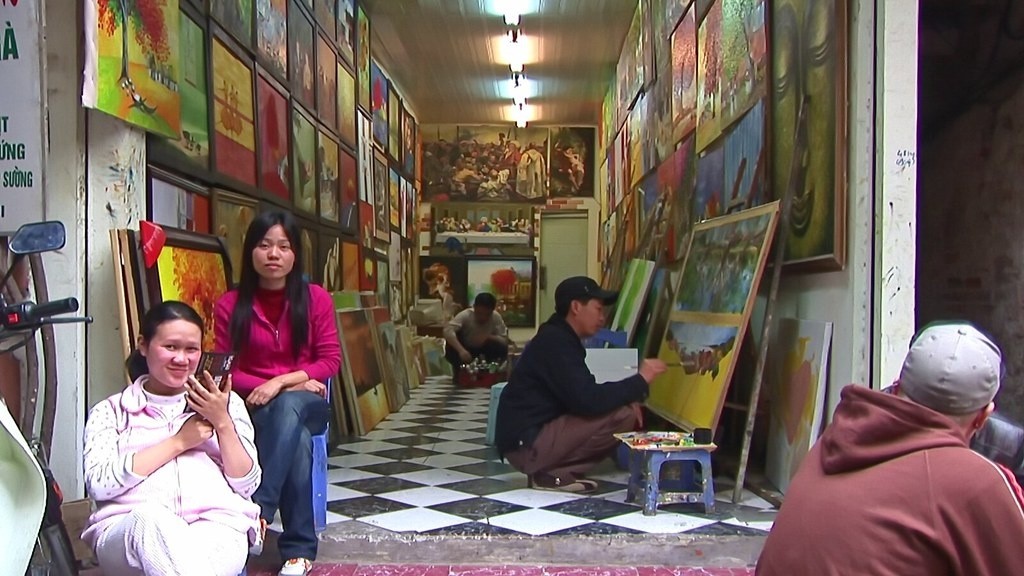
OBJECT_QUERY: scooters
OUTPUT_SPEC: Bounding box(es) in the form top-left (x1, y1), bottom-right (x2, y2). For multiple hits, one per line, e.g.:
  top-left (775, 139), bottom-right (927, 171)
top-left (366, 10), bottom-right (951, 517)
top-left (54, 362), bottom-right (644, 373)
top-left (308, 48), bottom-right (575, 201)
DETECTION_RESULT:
top-left (0, 219), bottom-right (94, 576)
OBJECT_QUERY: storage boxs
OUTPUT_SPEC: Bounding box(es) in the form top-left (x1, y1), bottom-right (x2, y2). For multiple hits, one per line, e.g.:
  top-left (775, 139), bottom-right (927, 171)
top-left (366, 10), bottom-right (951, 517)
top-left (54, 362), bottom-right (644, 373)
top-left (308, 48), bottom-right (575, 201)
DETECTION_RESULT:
top-left (456, 367), bottom-right (507, 389)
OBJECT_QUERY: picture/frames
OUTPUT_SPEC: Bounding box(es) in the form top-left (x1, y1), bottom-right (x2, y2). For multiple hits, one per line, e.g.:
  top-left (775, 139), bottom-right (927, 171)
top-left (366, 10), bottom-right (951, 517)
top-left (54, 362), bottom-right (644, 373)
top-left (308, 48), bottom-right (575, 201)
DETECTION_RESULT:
top-left (751, 0), bottom-right (847, 276)
top-left (111, 0), bottom-right (417, 385)
top-left (464, 256), bottom-right (536, 330)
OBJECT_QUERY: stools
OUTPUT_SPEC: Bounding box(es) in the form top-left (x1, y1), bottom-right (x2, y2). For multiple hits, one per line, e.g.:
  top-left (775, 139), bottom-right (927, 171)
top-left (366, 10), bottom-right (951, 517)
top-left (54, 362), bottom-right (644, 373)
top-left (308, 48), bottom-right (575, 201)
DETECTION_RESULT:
top-left (484, 381), bottom-right (508, 446)
top-left (623, 446), bottom-right (716, 515)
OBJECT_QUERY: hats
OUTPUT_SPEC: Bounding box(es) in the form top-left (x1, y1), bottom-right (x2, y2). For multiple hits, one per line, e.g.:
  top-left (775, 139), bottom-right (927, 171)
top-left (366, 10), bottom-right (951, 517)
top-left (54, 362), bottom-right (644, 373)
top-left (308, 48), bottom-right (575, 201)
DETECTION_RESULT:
top-left (910, 318), bottom-right (1007, 407)
top-left (899, 322), bottom-right (1001, 414)
top-left (554, 276), bottom-right (620, 309)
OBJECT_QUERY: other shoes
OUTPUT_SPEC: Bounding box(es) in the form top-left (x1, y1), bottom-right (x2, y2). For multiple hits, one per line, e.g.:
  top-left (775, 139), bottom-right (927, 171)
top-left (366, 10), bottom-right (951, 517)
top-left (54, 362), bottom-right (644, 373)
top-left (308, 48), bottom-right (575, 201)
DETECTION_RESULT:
top-left (531, 472), bottom-right (598, 493)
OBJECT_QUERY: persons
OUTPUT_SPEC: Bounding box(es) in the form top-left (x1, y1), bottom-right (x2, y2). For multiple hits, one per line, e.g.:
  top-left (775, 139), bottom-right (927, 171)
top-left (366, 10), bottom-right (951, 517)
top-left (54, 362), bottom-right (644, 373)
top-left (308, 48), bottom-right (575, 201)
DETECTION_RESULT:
top-left (444, 291), bottom-right (510, 387)
top-left (494, 275), bottom-right (667, 493)
top-left (212, 206), bottom-right (343, 575)
top-left (754, 319), bottom-right (1023, 576)
top-left (80, 300), bottom-right (262, 576)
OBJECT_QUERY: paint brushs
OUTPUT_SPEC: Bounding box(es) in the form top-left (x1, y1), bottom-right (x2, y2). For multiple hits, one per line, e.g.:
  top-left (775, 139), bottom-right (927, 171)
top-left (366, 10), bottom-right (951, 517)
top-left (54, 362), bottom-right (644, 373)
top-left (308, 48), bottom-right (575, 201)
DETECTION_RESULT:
top-left (624, 361), bottom-right (695, 372)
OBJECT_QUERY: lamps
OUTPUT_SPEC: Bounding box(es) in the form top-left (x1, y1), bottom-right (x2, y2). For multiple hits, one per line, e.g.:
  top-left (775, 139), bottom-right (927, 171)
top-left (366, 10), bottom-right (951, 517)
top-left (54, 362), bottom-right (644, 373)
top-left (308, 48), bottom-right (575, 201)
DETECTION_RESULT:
top-left (503, 12), bottom-right (525, 129)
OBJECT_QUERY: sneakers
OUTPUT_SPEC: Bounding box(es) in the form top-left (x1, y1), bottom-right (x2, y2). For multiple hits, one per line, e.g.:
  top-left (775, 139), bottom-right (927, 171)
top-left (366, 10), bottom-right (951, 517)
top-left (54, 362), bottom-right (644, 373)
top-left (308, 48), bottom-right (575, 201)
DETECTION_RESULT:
top-left (248, 518), bottom-right (267, 556)
top-left (278, 557), bottom-right (313, 576)
